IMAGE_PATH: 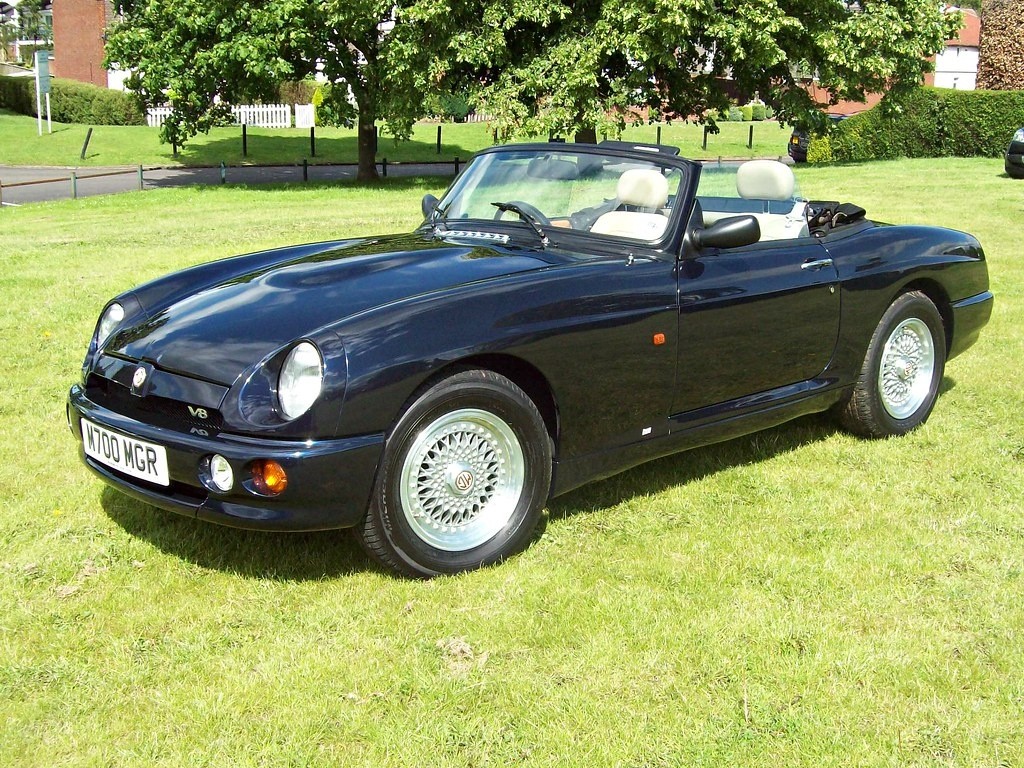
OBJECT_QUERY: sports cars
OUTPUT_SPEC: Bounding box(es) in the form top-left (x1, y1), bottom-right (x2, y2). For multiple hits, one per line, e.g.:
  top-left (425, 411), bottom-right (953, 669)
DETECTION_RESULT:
top-left (65, 139), bottom-right (995, 583)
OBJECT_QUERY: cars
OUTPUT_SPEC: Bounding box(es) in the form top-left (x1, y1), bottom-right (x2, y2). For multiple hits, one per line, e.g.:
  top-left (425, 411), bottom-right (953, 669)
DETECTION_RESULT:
top-left (786, 113), bottom-right (855, 164)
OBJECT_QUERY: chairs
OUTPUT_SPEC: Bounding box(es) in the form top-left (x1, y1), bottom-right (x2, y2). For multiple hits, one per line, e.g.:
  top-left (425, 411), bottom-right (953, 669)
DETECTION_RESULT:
top-left (721, 160), bottom-right (811, 242)
top-left (590, 169), bottom-right (668, 241)
